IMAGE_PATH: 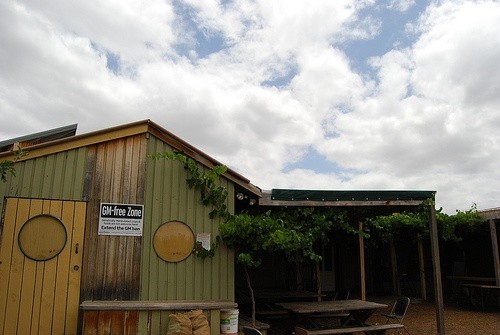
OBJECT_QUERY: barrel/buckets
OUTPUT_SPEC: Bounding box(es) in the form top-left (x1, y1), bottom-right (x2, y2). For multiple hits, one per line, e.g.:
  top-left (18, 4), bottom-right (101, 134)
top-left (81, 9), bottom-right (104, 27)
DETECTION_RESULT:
top-left (220, 309), bottom-right (239, 334)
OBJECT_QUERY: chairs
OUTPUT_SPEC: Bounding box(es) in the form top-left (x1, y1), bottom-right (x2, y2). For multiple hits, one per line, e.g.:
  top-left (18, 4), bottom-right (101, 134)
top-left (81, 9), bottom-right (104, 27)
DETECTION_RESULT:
top-left (241, 326), bottom-right (263, 335)
top-left (381, 296), bottom-right (412, 335)
top-left (333, 288), bottom-right (351, 314)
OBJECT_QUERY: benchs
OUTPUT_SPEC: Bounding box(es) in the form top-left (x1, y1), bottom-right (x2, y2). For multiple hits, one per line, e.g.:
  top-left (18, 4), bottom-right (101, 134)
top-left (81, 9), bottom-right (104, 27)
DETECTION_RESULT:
top-left (307, 324), bottom-right (404, 335)
top-left (256, 310), bottom-right (350, 327)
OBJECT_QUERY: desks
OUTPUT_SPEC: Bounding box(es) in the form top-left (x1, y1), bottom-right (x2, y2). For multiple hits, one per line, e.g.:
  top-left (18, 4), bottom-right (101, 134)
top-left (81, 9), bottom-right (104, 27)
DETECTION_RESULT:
top-left (81, 301), bottom-right (237, 312)
top-left (276, 300), bottom-right (388, 327)
top-left (243, 291), bottom-right (328, 311)
top-left (464, 284), bottom-right (500, 312)
